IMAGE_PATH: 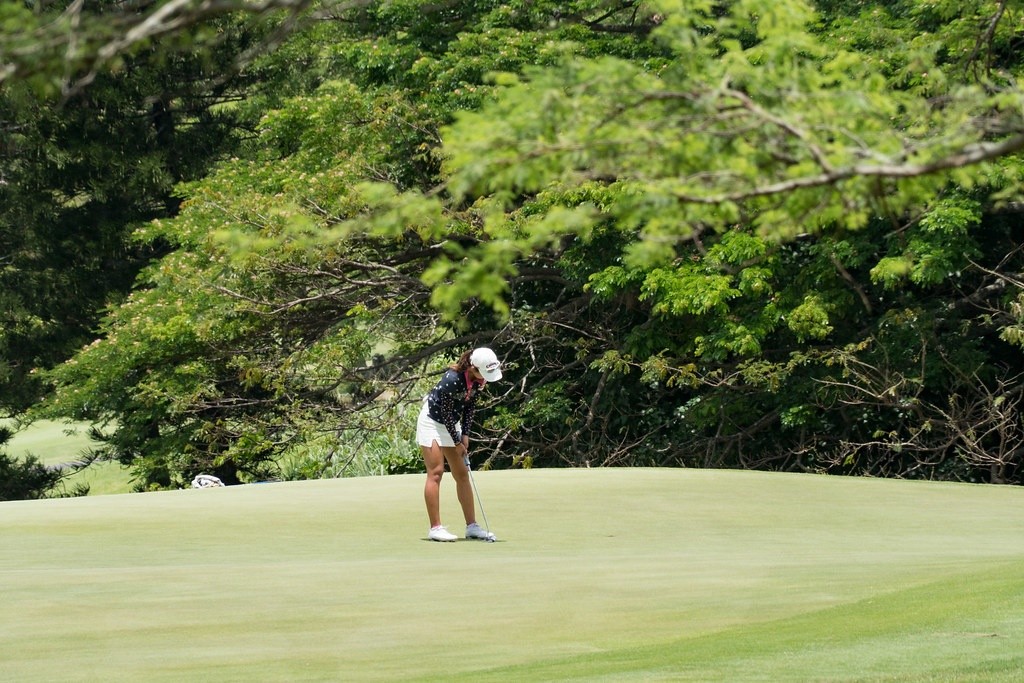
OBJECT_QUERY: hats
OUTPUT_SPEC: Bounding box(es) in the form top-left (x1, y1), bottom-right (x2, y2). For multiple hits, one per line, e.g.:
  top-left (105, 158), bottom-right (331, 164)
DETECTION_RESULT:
top-left (470, 347), bottom-right (503, 382)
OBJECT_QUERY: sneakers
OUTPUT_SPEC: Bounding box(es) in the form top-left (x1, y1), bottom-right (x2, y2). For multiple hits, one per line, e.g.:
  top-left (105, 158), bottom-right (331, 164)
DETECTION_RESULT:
top-left (428, 525), bottom-right (458, 542)
top-left (465, 523), bottom-right (494, 539)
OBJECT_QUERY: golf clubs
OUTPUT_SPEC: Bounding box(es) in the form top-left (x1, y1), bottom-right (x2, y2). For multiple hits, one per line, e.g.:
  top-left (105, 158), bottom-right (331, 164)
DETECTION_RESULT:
top-left (456, 430), bottom-right (496, 542)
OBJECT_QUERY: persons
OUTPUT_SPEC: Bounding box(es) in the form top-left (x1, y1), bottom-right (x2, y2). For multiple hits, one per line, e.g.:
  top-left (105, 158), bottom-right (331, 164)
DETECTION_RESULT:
top-left (416, 348), bottom-right (503, 542)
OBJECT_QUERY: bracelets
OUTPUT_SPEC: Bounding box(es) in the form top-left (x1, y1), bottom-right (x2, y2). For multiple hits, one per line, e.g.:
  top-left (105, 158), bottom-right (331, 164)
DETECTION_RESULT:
top-left (456, 442), bottom-right (460, 446)
